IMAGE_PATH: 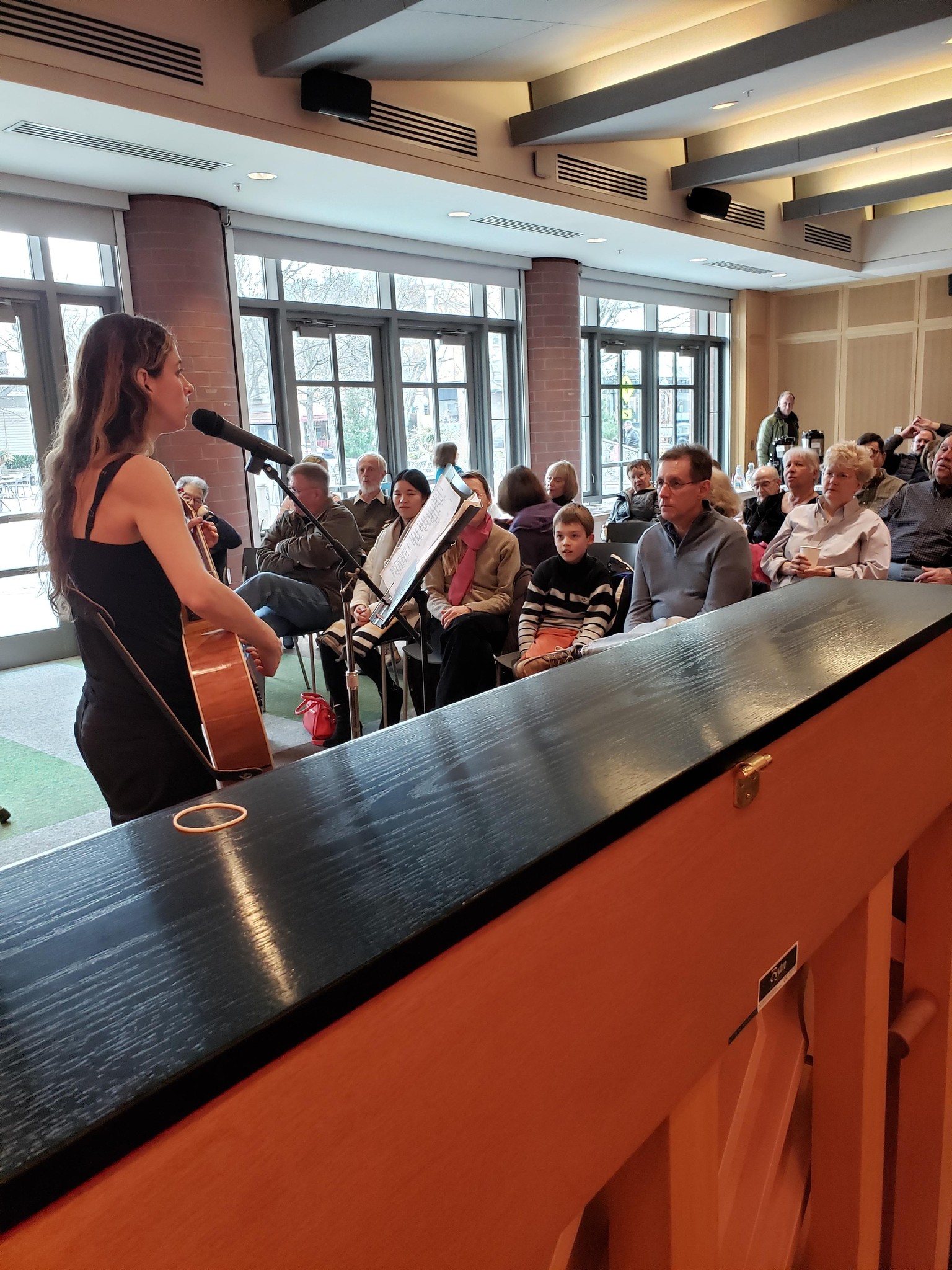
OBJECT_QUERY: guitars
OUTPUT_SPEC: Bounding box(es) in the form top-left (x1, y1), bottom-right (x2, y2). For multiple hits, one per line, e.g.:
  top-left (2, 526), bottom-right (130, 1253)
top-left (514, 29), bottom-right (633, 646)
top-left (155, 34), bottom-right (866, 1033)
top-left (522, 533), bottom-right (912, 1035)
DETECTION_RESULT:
top-left (180, 489), bottom-right (274, 790)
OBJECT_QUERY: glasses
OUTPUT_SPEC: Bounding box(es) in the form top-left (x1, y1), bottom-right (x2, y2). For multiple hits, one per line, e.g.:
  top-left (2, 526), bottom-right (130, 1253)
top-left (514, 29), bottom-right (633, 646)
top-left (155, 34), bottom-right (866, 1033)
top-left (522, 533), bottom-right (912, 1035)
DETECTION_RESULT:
top-left (286, 485), bottom-right (319, 494)
top-left (650, 480), bottom-right (703, 490)
top-left (821, 471), bottom-right (858, 479)
top-left (630, 471), bottom-right (649, 481)
top-left (751, 478), bottom-right (778, 492)
top-left (181, 493), bottom-right (203, 506)
top-left (871, 449), bottom-right (883, 457)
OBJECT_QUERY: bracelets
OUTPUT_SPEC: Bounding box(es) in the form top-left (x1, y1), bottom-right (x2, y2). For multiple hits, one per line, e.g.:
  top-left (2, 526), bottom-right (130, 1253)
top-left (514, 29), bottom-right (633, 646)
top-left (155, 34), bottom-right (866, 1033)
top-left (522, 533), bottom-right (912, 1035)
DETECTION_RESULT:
top-left (831, 569), bottom-right (835, 577)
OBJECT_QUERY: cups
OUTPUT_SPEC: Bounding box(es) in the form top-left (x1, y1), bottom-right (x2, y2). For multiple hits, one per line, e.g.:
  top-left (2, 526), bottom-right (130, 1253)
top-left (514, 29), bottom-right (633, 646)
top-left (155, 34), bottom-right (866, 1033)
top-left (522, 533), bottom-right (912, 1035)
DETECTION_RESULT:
top-left (800, 546), bottom-right (821, 570)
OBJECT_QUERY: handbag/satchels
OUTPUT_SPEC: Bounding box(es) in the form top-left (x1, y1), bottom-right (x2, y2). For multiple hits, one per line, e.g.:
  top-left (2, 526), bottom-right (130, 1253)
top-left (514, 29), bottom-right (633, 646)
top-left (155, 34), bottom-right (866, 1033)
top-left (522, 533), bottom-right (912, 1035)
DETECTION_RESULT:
top-left (294, 691), bottom-right (336, 746)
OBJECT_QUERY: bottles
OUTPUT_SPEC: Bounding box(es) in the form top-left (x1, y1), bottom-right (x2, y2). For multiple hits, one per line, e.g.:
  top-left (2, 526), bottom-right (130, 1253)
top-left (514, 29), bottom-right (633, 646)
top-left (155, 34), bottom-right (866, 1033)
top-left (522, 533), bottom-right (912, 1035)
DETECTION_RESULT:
top-left (734, 465), bottom-right (745, 491)
top-left (745, 462), bottom-right (755, 490)
top-left (767, 453), bottom-right (774, 467)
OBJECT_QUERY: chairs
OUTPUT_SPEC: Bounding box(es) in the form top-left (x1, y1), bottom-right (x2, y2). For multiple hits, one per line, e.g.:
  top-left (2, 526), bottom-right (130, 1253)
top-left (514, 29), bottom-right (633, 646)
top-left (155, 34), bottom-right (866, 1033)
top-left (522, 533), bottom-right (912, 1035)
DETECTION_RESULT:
top-left (238, 488), bottom-right (821, 728)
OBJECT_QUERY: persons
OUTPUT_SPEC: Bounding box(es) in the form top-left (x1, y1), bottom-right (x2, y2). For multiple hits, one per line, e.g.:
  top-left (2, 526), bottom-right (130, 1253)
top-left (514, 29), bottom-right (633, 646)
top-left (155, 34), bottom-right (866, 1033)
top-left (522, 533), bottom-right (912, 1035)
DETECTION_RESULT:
top-left (741, 466), bottom-right (785, 525)
top-left (518, 502), bottom-right (617, 661)
top-left (746, 446), bottom-right (823, 598)
top-left (315, 469), bottom-right (432, 748)
top-left (512, 444), bottom-right (752, 681)
top-left (420, 470), bottom-right (521, 711)
top-left (545, 459), bottom-right (578, 507)
top-left (337, 452), bottom-right (392, 556)
top-left (755, 391), bottom-right (799, 467)
top-left (34, 313), bottom-right (284, 828)
top-left (624, 420), bottom-right (640, 449)
top-left (175, 476), bottom-right (242, 622)
top-left (232, 462), bottom-right (367, 659)
top-left (853, 433), bottom-right (909, 515)
top-left (279, 454), bottom-right (341, 514)
top-left (877, 431), bottom-right (952, 584)
top-left (497, 464), bottom-right (562, 571)
top-left (880, 416), bottom-right (952, 484)
top-left (600, 459), bottom-right (661, 541)
top-left (433, 442), bottom-right (464, 483)
top-left (760, 440), bottom-right (892, 591)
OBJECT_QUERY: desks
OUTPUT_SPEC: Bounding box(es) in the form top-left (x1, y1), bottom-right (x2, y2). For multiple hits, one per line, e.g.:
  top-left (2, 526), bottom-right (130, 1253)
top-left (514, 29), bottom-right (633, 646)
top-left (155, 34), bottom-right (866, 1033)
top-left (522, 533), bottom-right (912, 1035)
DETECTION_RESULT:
top-left (733, 485), bottom-right (823, 519)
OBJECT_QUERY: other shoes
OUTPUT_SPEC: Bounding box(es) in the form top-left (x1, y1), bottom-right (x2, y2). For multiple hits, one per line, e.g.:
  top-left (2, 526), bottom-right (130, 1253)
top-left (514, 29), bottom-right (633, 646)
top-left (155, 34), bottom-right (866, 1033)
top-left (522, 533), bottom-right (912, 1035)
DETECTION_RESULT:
top-left (515, 641), bottom-right (577, 680)
top-left (377, 679), bottom-right (404, 731)
top-left (322, 711), bottom-right (363, 747)
top-left (283, 636), bottom-right (298, 648)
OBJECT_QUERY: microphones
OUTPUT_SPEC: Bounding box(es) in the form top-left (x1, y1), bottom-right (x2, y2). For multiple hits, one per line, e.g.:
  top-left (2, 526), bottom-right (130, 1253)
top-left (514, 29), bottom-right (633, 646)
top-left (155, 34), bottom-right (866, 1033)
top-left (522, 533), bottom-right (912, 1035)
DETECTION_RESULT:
top-left (190, 407), bottom-right (296, 467)
